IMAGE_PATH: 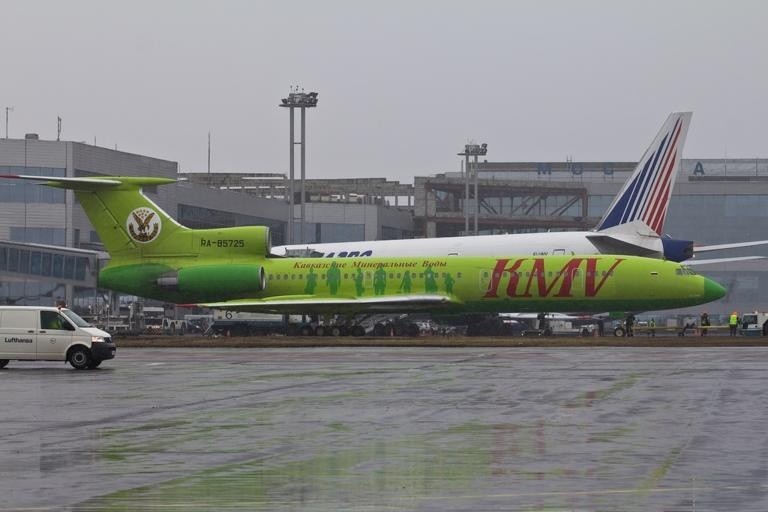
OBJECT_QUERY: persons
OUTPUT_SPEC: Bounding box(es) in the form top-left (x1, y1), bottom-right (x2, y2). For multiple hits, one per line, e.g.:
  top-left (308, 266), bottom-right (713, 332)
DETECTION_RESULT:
top-left (729, 311), bottom-right (740, 337)
top-left (742, 319), bottom-right (748, 329)
top-left (701, 313), bottom-right (711, 337)
top-left (691, 322), bottom-right (695, 329)
top-left (598, 316), bottom-right (605, 337)
top-left (626, 313), bottom-right (635, 337)
top-left (678, 323), bottom-right (688, 336)
top-left (650, 317), bottom-right (656, 336)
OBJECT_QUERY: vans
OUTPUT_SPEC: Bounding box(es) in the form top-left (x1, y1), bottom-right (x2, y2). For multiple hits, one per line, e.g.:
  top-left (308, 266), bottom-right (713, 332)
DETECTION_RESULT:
top-left (0, 306), bottom-right (116, 369)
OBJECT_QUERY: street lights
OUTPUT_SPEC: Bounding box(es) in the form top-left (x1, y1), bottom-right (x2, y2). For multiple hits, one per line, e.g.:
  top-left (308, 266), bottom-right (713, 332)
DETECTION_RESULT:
top-left (457, 142), bottom-right (486, 235)
top-left (279, 93), bottom-right (318, 322)
